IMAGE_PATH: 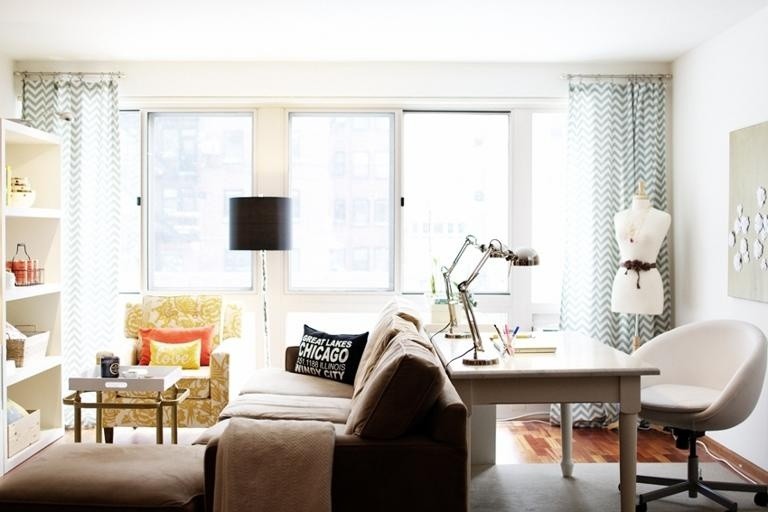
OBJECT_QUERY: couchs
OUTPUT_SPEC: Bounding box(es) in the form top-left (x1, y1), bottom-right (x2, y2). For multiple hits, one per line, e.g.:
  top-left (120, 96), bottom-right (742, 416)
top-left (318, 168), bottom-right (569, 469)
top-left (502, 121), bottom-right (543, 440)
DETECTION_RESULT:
top-left (96, 294), bottom-right (243, 442)
top-left (192, 327), bottom-right (468, 512)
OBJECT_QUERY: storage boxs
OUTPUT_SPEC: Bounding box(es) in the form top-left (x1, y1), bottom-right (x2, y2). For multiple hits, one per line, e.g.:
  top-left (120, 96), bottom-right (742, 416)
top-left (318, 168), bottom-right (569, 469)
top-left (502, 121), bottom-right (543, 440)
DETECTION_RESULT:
top-left (7, 402), bottom-right (41, 458)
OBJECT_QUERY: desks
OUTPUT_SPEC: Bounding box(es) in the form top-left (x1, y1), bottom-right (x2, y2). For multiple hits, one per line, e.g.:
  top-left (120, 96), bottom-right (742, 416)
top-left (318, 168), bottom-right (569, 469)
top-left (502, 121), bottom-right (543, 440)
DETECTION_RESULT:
top-left (429, 329), bottom-right (660, 512)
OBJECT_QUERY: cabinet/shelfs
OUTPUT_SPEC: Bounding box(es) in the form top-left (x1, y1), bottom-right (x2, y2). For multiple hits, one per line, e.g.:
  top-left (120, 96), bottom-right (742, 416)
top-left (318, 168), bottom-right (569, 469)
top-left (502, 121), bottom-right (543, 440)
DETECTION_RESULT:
top-left (0, 119), bottom-right (64, 478)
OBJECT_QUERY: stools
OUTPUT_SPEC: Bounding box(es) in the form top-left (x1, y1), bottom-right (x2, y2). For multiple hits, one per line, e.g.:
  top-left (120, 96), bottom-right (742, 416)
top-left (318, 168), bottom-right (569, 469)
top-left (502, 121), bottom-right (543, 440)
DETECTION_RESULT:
top-left (0, 443), bottom-right (205, 512)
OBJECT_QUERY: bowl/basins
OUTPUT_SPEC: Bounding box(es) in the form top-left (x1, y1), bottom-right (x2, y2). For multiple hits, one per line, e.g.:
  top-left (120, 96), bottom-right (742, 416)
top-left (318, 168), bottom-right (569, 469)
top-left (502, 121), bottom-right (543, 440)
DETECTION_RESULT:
top-left (11, 192), bottom-right (35, 208)
top-left (11, 177), bottom-right (27, 185)
top-left (12, 185), bottom-right (31, 191)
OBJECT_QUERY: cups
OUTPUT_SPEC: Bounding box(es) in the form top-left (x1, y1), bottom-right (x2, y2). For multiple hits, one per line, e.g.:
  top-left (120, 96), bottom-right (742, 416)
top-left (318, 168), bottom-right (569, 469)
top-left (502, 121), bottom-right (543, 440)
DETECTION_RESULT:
top-left (34, 260), bottom-right (39, 283)
top-left (6, 262), bottom-right (12, 268)
top-left (14, 261), bottom-right (28, 285)
top-left (100, 356), bottom-right (120, 378)
top-left (27, 260), bottom-right (34, 283)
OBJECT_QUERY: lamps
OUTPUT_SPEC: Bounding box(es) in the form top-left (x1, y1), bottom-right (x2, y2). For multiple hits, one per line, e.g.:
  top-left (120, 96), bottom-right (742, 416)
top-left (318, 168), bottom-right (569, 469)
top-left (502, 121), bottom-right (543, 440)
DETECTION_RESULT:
top-left (440, 234), bottom-right (508, 339)
top-left (457, 238), bottom-right (539, 365)
top-left (230, 196), bottom-right (293, 369)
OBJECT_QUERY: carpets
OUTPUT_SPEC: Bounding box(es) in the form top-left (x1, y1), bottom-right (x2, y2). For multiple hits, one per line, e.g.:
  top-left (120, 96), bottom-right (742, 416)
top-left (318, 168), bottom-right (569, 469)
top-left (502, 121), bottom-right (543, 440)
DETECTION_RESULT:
top-left (468, 463), bottom-right (768, 512)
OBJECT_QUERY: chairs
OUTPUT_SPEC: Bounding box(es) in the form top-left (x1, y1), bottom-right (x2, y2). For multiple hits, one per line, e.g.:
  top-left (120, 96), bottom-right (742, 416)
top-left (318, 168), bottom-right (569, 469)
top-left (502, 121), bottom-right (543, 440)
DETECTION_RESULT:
top-left (618, 318), bottom-right (768, 512)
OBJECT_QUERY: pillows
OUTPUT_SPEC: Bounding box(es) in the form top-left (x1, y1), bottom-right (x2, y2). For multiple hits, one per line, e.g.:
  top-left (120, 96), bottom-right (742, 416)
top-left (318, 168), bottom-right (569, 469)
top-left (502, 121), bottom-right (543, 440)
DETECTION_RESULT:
top-left (146, 340), bottom-right (203, 369)
top-left (344, 299), bottom-right (446, 443)
top-left (138, 327), bottom-right (214, 366)
top-left (137, 295), bottom-right (226, 366)
top-left (294, 324), bottom-right (368, 387)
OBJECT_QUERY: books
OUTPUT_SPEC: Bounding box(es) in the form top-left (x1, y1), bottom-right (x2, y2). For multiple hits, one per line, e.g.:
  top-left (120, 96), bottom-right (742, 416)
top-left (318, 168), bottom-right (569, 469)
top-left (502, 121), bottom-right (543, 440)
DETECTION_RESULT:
top-left (491, 337), bottom-right (557, 354)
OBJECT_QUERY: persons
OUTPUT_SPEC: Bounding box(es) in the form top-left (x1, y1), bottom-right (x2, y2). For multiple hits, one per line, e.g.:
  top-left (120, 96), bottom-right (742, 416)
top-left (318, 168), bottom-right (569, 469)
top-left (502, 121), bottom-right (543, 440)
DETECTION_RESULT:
top-left (611, 189), bottom-right (670, 318)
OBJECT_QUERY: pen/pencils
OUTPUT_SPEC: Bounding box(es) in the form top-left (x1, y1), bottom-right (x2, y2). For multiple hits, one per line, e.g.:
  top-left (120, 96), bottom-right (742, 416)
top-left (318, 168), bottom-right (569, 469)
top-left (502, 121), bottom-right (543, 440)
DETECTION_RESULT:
top-left (494, 324), bottom-right (511, 356)
top-left (501, 327), bottom-right (519, 356)
top-left (490, 334), bottom-right (534, 340)
top-left (505, 324), bottom-right (514, 355)
